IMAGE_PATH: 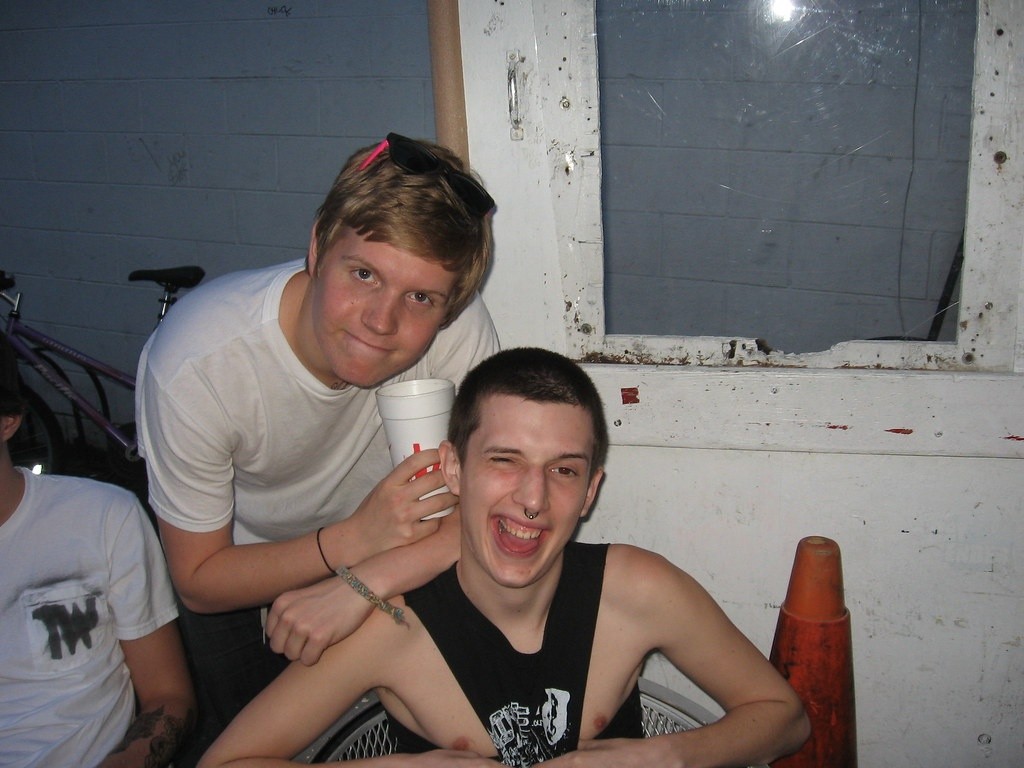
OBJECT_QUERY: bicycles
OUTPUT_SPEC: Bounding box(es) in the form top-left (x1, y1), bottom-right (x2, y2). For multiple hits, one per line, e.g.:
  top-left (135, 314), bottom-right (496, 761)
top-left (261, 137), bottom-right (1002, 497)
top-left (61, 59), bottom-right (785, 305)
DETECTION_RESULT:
top-left (0, 267), bottom-right (212, 510)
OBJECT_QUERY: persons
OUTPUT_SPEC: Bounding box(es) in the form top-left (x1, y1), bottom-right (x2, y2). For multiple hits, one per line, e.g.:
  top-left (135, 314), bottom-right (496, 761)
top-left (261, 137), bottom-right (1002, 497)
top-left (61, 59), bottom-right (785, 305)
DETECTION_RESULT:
top-left (0, 329), bottom-right (198, 768)
top-left (198, 347), bottom-right (812, 768)
top-left (136, 136), bottom-right (500, 767)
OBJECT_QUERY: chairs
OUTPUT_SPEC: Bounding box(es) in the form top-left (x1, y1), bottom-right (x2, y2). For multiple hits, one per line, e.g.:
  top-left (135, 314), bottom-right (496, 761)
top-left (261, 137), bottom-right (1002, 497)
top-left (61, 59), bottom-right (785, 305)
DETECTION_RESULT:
top-left (289, 678), bottom-right (769, 768)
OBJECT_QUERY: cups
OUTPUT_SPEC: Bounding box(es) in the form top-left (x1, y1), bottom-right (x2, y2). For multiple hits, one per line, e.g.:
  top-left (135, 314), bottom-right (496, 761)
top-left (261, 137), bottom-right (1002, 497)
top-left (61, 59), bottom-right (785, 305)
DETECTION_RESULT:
top-left (375, 378), bottom-right (457, 522)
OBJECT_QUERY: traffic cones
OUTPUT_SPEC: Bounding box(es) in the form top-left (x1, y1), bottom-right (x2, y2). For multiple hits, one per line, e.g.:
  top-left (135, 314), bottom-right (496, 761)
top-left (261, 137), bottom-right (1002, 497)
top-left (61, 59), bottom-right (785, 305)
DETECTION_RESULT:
top-left (759, 533), bottom-right (863, 768)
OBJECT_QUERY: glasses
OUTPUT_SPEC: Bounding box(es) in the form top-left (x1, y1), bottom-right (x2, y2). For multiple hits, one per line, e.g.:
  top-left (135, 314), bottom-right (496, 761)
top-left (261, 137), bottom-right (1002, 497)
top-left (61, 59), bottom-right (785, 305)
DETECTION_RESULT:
top-left (358, 132), bottom-right (495, 219)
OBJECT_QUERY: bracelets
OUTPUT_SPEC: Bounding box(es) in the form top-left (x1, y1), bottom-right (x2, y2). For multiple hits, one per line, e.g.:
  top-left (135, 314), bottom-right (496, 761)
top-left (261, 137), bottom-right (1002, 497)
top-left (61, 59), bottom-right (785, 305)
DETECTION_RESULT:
top-left (336, 567), bottom-right (411, 628)
top-left (317, 527), bottom-right (336, 573)
